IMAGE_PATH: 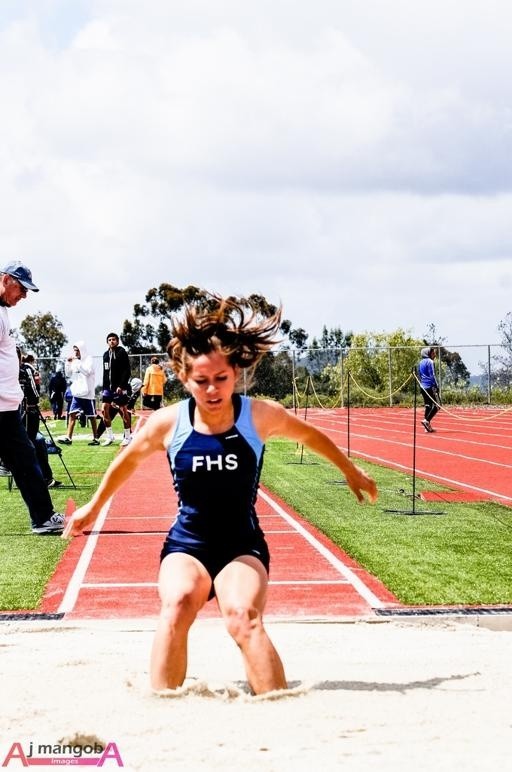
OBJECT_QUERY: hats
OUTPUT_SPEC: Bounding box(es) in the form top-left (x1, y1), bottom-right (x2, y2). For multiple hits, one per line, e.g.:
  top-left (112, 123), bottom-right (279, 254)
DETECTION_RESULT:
top-left (0, 260), bottom-right (40, 293)
top-left (130, 377), bottom-right (143, 393)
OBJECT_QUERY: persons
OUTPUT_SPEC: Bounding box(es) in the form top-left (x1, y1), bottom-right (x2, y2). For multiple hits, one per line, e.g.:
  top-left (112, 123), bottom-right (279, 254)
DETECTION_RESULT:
top-left (0, 259), bottom-right (70, 534)
top-left (15, 332), bottom-right (168, 488)
top-left (61, 290), bottom-right (377, 695)
top-left (418, 347), bottom-right (441, 432)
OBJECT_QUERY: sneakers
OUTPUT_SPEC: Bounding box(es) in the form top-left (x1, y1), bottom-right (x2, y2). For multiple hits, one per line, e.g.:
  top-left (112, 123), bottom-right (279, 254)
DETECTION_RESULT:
top-left (54, 416), bottom-right (61, 420)
top-left (421, 419), bottom-right (434, 433)
top-left (31, 513), bottom-right (65, 534)
top-left (120, 437), bottom-right (132, 446)
top-left (57, 437), bottom-right (72, 445)
top-left (88, 435), bottom-right (115, 447)
top-left (46, 478), bottom-right (64, 489)
top-left (0, 461), bottom-right (13, 476)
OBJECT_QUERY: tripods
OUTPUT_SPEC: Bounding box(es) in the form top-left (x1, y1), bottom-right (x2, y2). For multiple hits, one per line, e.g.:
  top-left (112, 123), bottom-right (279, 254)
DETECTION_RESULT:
top-left (7, 405), bottom-right (77, 491)
